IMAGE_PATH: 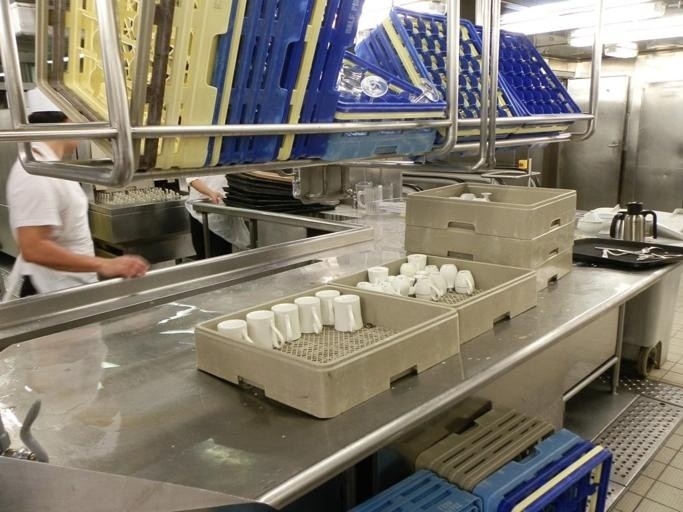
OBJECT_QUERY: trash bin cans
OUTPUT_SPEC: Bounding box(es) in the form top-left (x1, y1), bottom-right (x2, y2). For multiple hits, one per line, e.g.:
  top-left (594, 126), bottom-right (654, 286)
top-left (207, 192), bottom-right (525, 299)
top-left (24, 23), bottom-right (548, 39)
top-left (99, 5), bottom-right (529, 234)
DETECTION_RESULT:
top-left (621, 265), bottom-right (681, 378)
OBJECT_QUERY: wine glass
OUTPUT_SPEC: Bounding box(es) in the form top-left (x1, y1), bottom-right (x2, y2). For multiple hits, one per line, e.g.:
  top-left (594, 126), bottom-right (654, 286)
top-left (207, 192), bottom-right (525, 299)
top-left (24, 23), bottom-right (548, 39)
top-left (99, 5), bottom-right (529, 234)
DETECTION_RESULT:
top-left (361, 75), bottom-right (440, 104)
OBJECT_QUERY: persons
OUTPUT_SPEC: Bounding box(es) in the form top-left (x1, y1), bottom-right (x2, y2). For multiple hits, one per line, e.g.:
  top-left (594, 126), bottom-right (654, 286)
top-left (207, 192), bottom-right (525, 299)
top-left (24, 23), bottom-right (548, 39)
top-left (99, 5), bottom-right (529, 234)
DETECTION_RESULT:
top-left (4, 88), bottom-right (149, 304)
top-left (176, 174), bottom-right (250, 264)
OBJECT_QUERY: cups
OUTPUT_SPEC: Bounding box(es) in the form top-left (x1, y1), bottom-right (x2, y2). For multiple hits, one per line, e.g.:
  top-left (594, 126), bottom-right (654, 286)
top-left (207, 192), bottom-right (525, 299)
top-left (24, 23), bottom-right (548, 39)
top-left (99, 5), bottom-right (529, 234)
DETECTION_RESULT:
top-left (357, 253), bottom-right (476, 301)
top-left (335, 62), bottom-right (368, 105)
top-left (353, 181), bottom-right (382, 216)
top-left (216, 289), bottom-right (364, 351)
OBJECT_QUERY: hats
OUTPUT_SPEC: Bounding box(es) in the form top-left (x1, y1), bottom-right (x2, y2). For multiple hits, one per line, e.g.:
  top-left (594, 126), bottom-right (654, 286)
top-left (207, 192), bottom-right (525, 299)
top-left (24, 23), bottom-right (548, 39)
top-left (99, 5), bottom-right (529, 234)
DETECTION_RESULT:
top-left (25, 88), bottom-right (65, 113)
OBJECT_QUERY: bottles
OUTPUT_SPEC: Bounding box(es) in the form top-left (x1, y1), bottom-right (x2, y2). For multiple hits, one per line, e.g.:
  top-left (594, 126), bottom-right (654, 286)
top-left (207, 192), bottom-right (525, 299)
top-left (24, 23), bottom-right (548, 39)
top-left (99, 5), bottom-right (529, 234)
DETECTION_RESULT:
top-left (610, 201), bottom-right (658, 243)
